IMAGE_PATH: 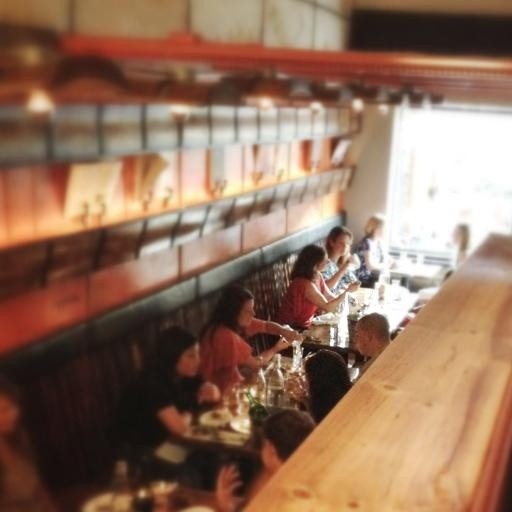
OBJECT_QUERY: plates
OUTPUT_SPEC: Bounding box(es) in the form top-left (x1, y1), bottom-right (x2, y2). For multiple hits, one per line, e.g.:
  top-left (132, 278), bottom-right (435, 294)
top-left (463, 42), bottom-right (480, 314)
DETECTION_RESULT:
top-left (199, 409), bottom-right (251, 435)
top-left (83, 490), bottom-right (131, 512)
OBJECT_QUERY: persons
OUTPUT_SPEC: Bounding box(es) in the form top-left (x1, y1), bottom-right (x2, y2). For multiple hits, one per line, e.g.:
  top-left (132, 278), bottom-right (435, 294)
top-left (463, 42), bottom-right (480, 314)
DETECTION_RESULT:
top-left (121, 326), bottom-right (222, 489)
top-left (268, 243), bottom-right (362, 359)
top-left (408, 270), bottom-right (453, 314)
top-left (350, 313), bottom-right (392, 384)
top-left (319, 224), bottom-right (359, 294)
top-left (197, 283), bottom-right (303, 396)
top-left (451, 223), bottom-right (470, 265)
top-left (352, 212), bottom-right (397, 289)
top-left (287, 348), bottom-right (353, 425)
top-left (215, 409), bottom-right (316, 512)
top-left (1, 375), bottom-right (62, 511)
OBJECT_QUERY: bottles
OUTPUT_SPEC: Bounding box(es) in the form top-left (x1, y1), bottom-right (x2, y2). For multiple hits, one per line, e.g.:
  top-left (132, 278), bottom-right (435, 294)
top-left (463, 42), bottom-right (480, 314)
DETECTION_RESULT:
top-left (242, 354), bottom-right (284, 424)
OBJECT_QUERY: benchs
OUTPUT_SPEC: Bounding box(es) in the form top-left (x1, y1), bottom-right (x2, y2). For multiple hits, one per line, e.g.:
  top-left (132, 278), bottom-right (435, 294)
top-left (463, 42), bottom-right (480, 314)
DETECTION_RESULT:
top-left (1, 239), bottom-right (327, 512)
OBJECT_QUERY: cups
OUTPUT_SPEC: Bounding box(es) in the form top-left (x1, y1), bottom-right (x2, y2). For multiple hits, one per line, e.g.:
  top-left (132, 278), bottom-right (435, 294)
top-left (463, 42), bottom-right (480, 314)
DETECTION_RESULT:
top-left (329, 323), bottom-right (339, 340)
top-left (293, 346), bottom-right (303, 371)
